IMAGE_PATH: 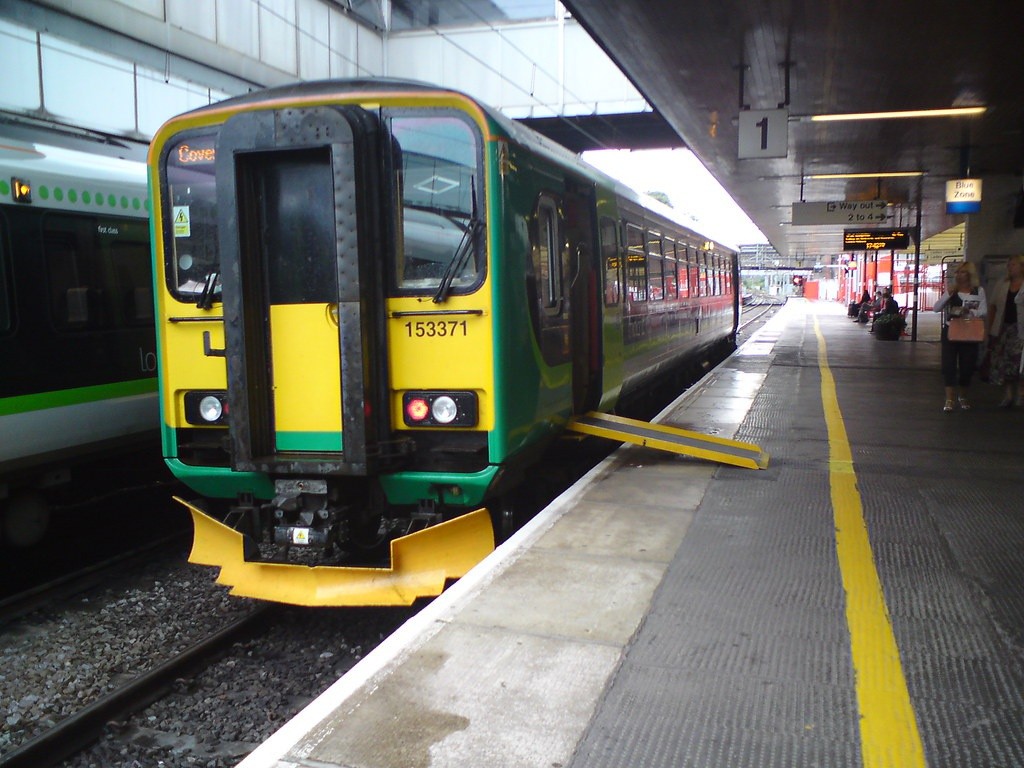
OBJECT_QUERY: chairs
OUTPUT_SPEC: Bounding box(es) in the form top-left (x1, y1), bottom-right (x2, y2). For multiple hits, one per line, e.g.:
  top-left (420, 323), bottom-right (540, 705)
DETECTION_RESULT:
top-left (898, 306), bottom-right (914, 337)
top-left (863, 298), bottom-right (886, 325)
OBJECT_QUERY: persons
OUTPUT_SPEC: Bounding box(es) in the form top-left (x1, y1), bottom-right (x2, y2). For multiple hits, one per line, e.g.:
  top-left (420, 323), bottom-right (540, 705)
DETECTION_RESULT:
top-left (857, 290), bottom-right (870, 305)
top-left (934, 261), bottom-right (986, 411)
top-left (852, 291), bottom-right (882, 322)
top-left (868, 292), bottom-right (899, 332)
top-left (987, 254), bottom-right (1024, 408)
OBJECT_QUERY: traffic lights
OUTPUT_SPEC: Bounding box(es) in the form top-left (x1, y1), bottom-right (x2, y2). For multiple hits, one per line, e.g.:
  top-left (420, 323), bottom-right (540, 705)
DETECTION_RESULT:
top-left (792, 275), bottom-right (803, 286)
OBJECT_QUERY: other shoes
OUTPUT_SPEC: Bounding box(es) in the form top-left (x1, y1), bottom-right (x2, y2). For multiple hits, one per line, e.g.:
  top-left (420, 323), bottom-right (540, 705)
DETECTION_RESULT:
top-left (958, 397), bottom-right (971, 409)
top-left (853, 320), bottom-right (861, 323)
top-left (869, 328), bottom-right (873, 333)
top-left (944, 400), bottom-right (955, 411)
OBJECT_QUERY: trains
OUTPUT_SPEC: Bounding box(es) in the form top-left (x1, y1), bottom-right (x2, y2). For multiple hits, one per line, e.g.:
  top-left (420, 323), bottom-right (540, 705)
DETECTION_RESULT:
top-left (0, 138), bottom-right (476, 500)
top-left (148, 76), bottom-right (743, 603)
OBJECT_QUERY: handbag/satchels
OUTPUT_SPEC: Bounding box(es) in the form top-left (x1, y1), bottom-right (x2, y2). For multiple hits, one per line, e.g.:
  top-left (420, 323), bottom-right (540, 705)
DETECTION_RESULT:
top-left (947, 310), bottom-right (984, 340)
